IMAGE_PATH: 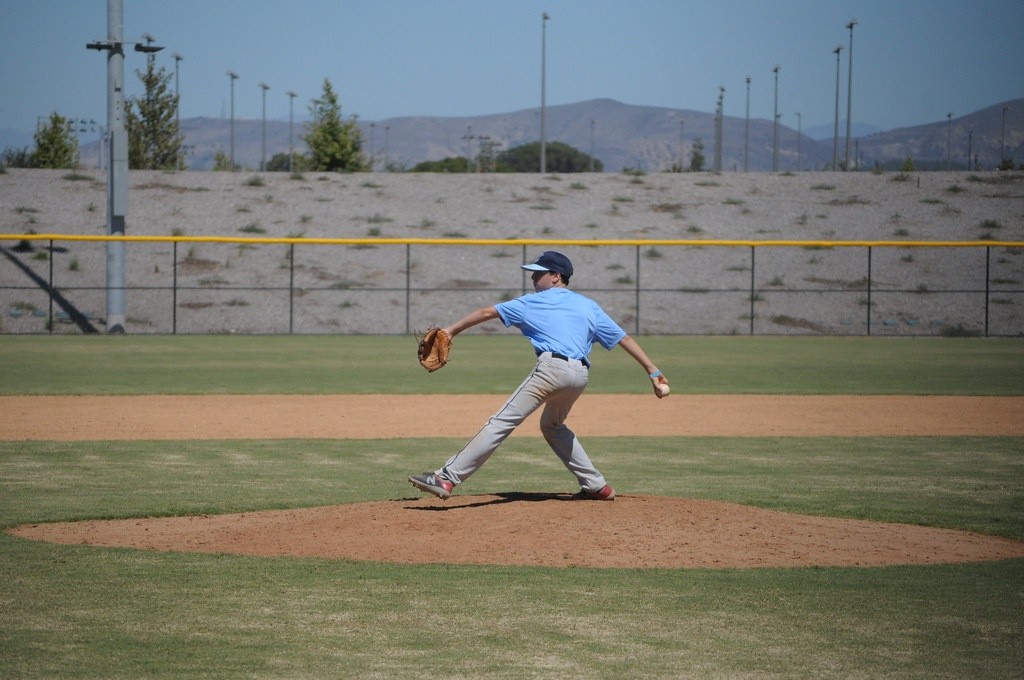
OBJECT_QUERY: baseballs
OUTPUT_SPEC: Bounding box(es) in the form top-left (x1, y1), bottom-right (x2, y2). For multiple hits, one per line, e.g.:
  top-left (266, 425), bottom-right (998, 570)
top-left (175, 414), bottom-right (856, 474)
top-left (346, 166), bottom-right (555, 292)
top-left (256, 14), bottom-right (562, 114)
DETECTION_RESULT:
top-left (658, 384), bottom-right (670, 396)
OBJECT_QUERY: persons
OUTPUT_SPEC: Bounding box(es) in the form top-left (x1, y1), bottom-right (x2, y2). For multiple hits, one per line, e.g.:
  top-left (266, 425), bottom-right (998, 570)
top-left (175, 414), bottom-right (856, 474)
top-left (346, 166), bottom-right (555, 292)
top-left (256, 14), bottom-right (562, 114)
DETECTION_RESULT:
top-left (409, 252), bottom-right (669, 500)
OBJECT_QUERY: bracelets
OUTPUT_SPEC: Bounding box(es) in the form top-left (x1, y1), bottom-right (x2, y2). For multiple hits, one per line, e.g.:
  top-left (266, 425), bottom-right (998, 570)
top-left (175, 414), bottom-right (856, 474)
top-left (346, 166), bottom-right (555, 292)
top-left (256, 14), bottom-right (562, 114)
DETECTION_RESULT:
top-left (648, 370), bottom-right (661, 379)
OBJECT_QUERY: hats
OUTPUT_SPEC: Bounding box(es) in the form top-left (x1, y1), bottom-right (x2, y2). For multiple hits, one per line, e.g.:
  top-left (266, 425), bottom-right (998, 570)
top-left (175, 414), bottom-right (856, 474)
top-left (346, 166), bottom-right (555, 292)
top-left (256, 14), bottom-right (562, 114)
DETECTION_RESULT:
top-left (521, 251), bottom-right (574, 279)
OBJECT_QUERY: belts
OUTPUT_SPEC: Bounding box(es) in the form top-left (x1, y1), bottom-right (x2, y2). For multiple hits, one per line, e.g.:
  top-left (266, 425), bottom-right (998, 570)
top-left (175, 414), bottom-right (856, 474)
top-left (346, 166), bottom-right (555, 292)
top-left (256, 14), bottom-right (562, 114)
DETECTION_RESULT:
top-left (537, 352), bottom-right (586, 366)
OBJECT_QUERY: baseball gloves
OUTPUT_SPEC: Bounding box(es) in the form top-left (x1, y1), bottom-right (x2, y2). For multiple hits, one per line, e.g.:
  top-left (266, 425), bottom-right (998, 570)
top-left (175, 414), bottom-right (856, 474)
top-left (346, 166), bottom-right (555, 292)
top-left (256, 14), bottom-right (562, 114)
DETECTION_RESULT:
top-left (414, 323), bottom-right (453, 373)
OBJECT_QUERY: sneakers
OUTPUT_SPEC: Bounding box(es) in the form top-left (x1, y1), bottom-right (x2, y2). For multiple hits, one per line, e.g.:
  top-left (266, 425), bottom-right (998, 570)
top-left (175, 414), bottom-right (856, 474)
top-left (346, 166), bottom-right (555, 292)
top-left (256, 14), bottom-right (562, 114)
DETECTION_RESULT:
top-left (408, 472), bottom-right (454, 500)
top-left (571, 484), bottom-right (615, 500)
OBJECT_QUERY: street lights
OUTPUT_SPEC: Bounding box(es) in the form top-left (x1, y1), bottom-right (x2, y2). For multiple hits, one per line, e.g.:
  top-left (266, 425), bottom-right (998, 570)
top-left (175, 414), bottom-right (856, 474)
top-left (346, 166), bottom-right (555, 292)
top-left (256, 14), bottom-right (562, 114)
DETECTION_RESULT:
top-left (384, 124), bottom-right (390, 172)
top-left (832, 43), bottom-right (844, 172)
top-left (946, 112), bottom-right (954, 172)
top-left (719, 85), bottom-right (725, 175)
top-left (370, 123), bottom-right (374, 172)
top-left (170, 52), bottom-right (184, 171)
top-left (286, 90), bottom-right (299, 172)
top-left (773, 64), bottom-right (779, 172)
top-left (540, 11), bottom-right (552, 190)
top-left (843, 17), bottom-right (860, 171)
top-left (1000, 106), bottom-right (1009, 171)
top-left (224, 70), bottom-right (238, 171)
top-left (744, 76), bottom-right (752, 172)
top-left (259, 80), bottom-right (270, 172)
top-left (140, 32), bottom-right (155, 168)
top-left (795, 112), bottom-right (802, 171)
